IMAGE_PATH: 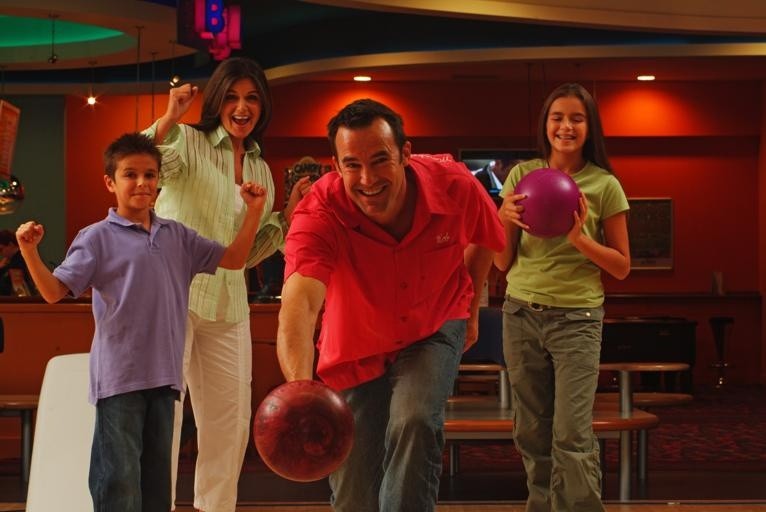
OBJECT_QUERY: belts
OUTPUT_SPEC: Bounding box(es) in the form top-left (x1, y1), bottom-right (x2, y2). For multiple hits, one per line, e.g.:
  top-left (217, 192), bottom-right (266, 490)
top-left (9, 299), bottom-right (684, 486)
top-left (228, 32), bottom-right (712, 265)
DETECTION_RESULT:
top-left (506, 295), bottom-right (554, 312)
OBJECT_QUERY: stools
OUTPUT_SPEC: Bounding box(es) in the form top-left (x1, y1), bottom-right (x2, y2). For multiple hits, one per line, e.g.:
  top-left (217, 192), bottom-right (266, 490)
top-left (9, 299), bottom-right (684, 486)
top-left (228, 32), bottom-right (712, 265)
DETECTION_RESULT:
top-left (708, 313), bottom-right (736, 392)
top-left (1, 392), bottom-right (39, 500)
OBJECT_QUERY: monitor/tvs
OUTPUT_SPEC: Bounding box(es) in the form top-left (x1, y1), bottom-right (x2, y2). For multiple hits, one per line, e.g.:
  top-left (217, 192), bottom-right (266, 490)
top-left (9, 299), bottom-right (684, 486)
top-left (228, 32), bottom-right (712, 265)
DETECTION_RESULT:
top-left (459, 148), bottom-right (542, 199)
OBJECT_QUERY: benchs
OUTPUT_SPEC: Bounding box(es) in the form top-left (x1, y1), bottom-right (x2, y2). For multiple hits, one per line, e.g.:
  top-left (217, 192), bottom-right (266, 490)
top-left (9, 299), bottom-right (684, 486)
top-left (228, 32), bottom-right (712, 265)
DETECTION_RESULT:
top-left (443, 392), bottom-right (693, 477)
top-left (442, 406), bottom-right (659, 502)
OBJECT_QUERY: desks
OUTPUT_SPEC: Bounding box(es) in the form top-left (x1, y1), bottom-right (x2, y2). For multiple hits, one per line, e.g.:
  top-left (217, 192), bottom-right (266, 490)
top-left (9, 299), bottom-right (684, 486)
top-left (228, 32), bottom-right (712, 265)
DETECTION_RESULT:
top-left (442, 358), bottom-right (695, 497)
top-left (1, 295), bottom-right (281, 436)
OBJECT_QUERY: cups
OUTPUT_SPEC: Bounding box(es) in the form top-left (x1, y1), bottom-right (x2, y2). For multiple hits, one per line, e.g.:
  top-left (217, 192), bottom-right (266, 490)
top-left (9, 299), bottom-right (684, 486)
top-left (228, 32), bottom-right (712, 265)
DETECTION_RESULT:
top-left (710, 271), bottom-right (723, 297)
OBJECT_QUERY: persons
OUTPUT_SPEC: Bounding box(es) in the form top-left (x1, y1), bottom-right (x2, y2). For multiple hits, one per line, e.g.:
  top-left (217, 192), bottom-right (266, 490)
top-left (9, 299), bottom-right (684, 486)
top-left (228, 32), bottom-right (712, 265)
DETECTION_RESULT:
top-left (0, 228), bottom-right (36, 297)
top-left (496, 85), bottom-right (634, 511)
top-left (277, 98), bottom-right (501, 512)
top-left (141, 58), bottom-right (312, 512)
top-left (15, 132), bottom-right (269, 511)
